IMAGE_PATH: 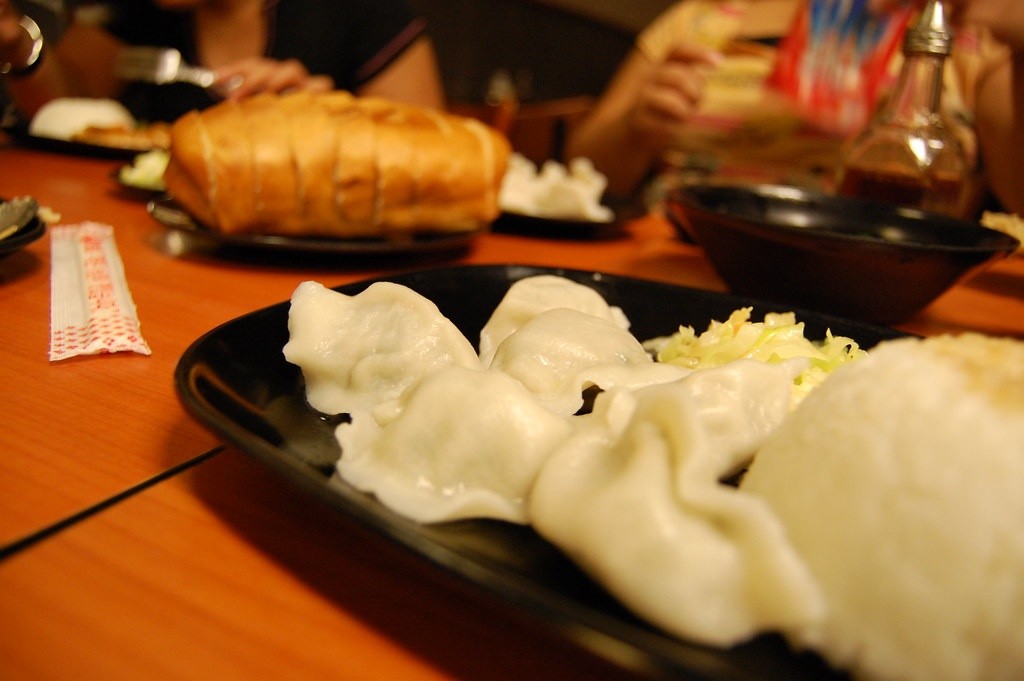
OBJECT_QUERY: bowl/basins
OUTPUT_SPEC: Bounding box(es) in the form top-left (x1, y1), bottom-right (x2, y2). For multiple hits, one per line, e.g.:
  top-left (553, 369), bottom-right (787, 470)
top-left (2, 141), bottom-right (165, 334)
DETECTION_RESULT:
top-left (669, 177), bottom-right (1022, 323)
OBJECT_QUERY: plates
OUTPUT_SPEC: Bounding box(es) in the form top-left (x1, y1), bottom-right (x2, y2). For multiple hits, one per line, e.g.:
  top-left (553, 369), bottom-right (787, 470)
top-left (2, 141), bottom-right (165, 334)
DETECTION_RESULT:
top-left (2, 123), bottom-right (150, 159)
top-left (0, 194), bottom-right (45, 254)
top-left (496, 205), bottom-right (616, 239)
top-left (170, 258), bottom-right (932, 680)
top-left (147, 191), bottom-right (479, 261)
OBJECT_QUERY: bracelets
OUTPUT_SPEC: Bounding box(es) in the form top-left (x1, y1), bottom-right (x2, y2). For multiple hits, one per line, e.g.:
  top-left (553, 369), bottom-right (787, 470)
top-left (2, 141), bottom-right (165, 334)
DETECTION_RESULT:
top-left (5, 17), bottom-right (44, 75)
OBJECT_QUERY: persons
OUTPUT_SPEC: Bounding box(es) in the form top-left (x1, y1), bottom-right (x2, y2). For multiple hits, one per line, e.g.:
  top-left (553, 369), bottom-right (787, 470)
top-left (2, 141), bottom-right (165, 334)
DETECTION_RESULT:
top-left (571, 0), bottom-right (1024, 220)
top-left (0, 0), bottom-right (448, 119)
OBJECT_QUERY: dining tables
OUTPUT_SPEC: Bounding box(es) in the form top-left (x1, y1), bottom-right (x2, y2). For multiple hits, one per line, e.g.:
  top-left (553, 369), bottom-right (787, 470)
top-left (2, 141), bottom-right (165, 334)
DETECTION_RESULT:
top-left (0, 139), bottom-right (1024, 681)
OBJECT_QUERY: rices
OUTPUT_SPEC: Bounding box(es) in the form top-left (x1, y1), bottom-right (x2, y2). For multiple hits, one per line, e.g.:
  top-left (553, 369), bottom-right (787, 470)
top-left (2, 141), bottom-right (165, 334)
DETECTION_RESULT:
top-left (734, 331), bottom-right (1024, 681)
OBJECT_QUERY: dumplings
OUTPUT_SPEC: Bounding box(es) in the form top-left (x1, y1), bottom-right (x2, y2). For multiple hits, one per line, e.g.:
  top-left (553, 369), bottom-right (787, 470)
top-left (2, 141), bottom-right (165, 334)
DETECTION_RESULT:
top-left (494, 149), bottom-right (615, 223)
top-left (281, 269), bottom-right (824, 648)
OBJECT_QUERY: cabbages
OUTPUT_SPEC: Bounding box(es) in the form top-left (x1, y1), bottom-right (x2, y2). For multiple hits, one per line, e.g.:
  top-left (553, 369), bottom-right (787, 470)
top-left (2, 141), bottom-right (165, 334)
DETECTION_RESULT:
top-left (644, 307), bottom-right (868, 407)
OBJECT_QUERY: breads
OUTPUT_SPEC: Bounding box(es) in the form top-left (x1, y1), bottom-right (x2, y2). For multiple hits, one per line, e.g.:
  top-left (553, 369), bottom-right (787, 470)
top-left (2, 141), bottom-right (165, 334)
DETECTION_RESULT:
top-left (164, 89), bottom-right (512, 235)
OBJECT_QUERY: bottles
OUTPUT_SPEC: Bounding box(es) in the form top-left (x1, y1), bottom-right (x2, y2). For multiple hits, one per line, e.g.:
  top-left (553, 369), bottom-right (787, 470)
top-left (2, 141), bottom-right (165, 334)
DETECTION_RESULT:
top-left (825, 0), bottom-right (982, 218)
